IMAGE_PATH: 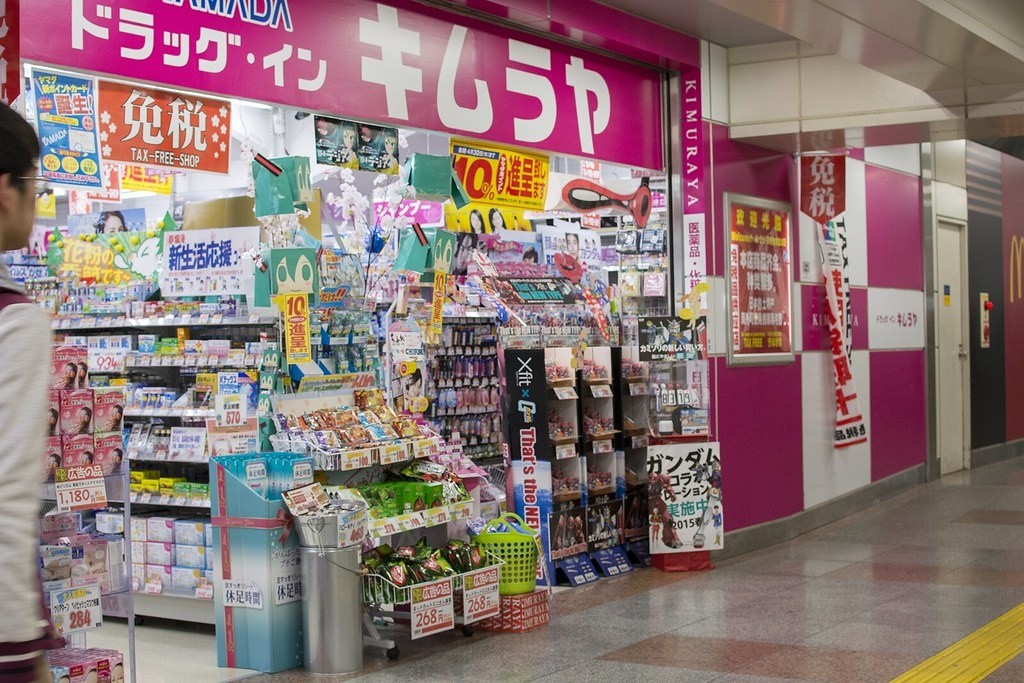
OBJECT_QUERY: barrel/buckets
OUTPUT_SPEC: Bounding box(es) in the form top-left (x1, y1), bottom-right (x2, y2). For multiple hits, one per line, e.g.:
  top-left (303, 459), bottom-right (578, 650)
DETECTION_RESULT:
top-left (299, 544), bottom-right (369, 675)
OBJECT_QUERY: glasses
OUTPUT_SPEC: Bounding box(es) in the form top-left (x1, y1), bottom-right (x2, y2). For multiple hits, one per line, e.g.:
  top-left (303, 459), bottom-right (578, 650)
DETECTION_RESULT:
top-left (16, 175), bottom-right (50, 194)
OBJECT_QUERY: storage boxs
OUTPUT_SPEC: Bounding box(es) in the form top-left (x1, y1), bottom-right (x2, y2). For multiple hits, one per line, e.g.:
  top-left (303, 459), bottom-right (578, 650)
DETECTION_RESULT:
top-left (65, 335), bottom-right (87, 345)
top-left (138, 335), bottom-right (159, 353)
top-left (175, 545), bottom-right (207, 569)
top-left (130, 515), bottom-right (147, 542)
top-left (146, 542), bottom-right (176, 567)
top-left (62, 433), bottom-right (95, 468)
top-left (59, 388), bottom-right (93, 435)
top-left (184, 340), bottom-right (209, 356)
top-left (131, 563), bottom-right (146, 586)
top-left (110, 335), bottom-right (137, 350)
top-left (171, 566), bottom-right (205, 590)
top-left (146, 564), bottom-right (172, 590)
top-left (93, 431), bottom-right (122, 474)
top-left (87, 387), bottom-right (124, 433)
top-left (40, 435), bottom-right (62, 483)
top-left (131, 542), bottom-right (146, 564)
top-left (174, 520), bottom-right (206, 546)
top-left (146, 517), bottom-right (177, 544)
top-left (88, 336), bottom-right (110, 351)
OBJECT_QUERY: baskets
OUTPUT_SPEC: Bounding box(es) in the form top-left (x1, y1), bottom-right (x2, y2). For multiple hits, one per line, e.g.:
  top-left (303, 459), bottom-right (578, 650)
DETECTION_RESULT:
top-left (472, 512), bottom-right (537, 595)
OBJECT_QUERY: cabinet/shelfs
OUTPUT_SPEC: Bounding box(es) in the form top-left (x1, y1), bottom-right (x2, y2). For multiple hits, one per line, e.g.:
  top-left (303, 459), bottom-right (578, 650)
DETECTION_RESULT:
top-left (36, 197), bottom-right (667, 683)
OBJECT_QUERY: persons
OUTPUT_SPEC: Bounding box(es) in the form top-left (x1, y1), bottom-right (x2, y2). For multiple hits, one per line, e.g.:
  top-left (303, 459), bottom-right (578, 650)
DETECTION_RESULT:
top-left (565, 233), bottom-right (579, 260)
top-left (405, 368), bottom-right (422, 400)
top-left (84, 668), bottom-right (97, 683)
top-left (452, 234), bottom-right (479, 277)
top-left (46, 361), bottom-right (123, 480)
top-left (0, 102), bottom-right (53, 683)
top-left (583, 238), bottom-right (601, 260)
top-left (111, 662), bottom-right (124, 683)
top-left (488, 208), bottom-right (507, 235)
top-left (523, 248), bottom-right (539, 263)
top-left (469, 209), bottom-right (486, 234)
top-left (93, 211), bottom-right (125, 233)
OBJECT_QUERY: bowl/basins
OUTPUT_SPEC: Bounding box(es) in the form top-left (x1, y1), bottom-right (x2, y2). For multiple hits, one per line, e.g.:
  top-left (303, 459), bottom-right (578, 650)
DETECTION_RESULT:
top-left (291, 499), bottom-right (368, 548)
